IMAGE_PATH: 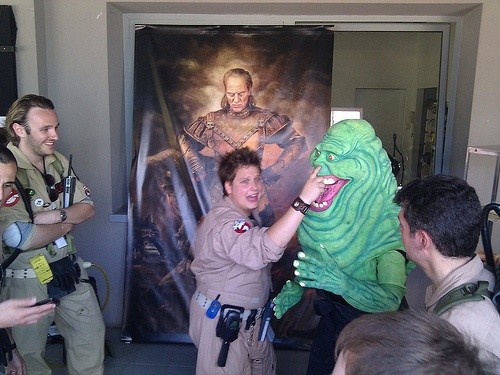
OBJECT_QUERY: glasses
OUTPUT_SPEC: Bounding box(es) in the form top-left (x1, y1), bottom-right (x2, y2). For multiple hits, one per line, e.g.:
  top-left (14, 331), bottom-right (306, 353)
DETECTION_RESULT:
top-left (44, 174), bottom-right (59, 202)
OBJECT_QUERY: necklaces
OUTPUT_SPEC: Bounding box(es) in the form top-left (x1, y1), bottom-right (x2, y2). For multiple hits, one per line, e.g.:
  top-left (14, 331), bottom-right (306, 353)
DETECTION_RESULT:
top-left (31, 156), bottom-right (48, 180)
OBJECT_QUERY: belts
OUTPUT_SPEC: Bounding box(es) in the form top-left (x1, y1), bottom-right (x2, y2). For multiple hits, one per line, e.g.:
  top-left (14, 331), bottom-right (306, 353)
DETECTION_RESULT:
top-left (193, 289), bottom-right (265, 319)
top-left (1, 254), bottom-right (78, 278)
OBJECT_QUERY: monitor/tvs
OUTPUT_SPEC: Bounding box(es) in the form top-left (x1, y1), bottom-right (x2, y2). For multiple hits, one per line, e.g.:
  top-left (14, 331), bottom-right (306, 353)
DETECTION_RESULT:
top-left (330, 106), bottom-right (363, 128)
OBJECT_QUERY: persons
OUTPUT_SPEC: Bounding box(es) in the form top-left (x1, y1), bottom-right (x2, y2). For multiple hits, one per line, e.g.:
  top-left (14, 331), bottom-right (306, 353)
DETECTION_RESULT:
top-left (0, 145), bottom-right (56, 374)
top-left (188, 148), bottom-right (325, 375)
top-left (331, 310), bottom-right (485, 375)
top-left (393, 174), bottom-right (500, 375)
top-left (180, 68), bottom-right (308, 228)
top-left (0, 94), bottom-right (105, 375)
top-left (270, 119), bottom-right (416, 374)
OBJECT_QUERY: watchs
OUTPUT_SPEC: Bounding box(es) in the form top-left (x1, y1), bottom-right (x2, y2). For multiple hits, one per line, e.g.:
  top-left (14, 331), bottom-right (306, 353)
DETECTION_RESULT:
top-left (56, 208), bottom-right (68, 224)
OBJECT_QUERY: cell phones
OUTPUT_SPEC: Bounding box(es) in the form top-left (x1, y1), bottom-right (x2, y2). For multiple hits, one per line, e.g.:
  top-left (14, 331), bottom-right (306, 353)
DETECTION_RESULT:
top-left (28, 297), bottom-right (60, 308)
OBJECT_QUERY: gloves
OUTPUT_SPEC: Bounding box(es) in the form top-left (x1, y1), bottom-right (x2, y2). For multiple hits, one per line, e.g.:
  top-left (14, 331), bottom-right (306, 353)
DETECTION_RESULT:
top-left (47, 256), bottom-right (80, 292)
top-left (0, 328), bottom-right (13, 367)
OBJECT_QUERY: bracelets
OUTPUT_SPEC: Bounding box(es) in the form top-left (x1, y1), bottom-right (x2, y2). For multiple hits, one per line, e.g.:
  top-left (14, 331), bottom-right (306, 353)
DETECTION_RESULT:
top-left (291, 197), bottom-right (312, 216)
